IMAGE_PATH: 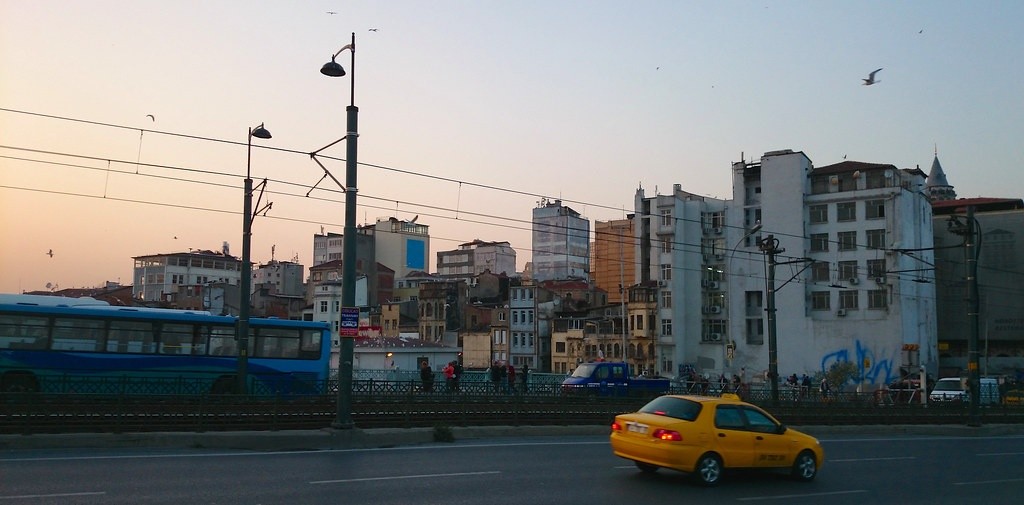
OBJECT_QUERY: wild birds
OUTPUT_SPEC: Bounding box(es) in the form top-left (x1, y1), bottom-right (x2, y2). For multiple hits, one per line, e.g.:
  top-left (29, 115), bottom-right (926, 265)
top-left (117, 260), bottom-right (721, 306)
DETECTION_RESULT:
top-left (46, 249), bottom-right (54, 258)
top-left (861, 68), bottom-right (883, 85)
top-left (405, 215), bottom-right (418, 227)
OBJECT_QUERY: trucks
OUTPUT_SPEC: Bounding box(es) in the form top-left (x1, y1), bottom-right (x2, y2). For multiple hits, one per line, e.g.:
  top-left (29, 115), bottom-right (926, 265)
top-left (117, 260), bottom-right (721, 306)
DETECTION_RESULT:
top-left (563, 360), bottom-right (671, 402)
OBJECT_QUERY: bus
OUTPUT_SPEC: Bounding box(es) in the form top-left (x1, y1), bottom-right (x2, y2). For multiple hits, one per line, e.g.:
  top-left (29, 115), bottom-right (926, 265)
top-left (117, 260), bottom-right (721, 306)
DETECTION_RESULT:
top-left (0, 290), bottom-right (332, 404)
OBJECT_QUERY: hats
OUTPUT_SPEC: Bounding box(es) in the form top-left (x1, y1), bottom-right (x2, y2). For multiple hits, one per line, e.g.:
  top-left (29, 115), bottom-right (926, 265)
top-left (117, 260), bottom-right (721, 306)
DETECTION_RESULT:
top-left (493, 361), bottom-right (498, 364)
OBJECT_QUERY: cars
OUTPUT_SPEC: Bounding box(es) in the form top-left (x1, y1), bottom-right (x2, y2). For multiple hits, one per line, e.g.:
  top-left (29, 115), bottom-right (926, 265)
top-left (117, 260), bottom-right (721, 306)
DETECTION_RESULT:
top-left (610, 394), bottom-right (821, 488)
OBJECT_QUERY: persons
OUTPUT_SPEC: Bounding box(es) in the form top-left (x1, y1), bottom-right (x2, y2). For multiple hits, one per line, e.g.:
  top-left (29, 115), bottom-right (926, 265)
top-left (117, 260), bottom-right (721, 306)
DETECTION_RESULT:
top-left (416, 354), bottom-right (532, 399)
top-left (630, 363), bottom-right (836, 406)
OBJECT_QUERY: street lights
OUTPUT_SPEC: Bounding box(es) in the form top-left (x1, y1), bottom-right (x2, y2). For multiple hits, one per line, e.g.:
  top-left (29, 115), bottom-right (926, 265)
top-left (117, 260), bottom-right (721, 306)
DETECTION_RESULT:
top-left (727, 223), bottom-right (756, 383)
top-left (321, 33), bottom-right (359, 429)
top-left (233, 124), bottom-right (276, 401)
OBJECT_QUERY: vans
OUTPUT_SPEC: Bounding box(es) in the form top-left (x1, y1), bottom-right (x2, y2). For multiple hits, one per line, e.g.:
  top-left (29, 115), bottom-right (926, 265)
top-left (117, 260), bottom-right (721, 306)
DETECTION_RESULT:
top-left (932, 376), bottom-right (973, 405)
